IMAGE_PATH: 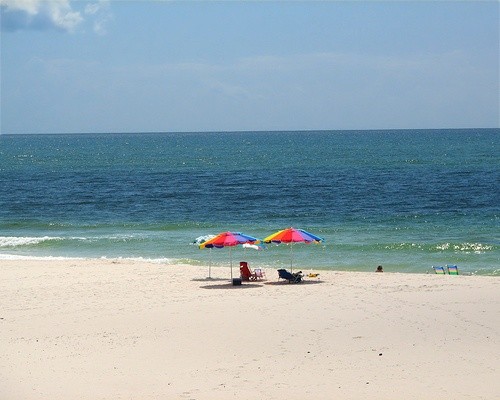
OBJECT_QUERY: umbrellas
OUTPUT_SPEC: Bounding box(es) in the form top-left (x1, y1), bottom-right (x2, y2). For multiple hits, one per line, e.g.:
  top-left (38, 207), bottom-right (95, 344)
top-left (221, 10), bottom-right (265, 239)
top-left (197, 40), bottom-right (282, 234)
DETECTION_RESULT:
top-left (198, 229), bottom-right (263, 285)
top-left (189, 233), bottom-right (224, 279)
top-left (262, 226), bottom-right (324, 274)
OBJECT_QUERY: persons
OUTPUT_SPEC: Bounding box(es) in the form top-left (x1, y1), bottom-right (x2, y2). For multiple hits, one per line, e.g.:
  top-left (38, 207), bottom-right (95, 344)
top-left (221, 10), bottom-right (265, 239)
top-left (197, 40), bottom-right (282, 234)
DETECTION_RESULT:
top-left (376, 265), bottom-right (383, 272)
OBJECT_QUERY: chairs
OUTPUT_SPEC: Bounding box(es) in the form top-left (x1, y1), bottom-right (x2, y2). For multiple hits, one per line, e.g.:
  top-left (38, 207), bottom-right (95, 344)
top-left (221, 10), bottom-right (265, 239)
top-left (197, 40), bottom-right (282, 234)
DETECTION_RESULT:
top-left (432, 265), bottom-right (445, 274)
top-left (446, 264), bottom-right (470, 276)
top-left (277, 269), bottom-right (304, 284)
top-left (239, 262), bottom-right (266, 280)
